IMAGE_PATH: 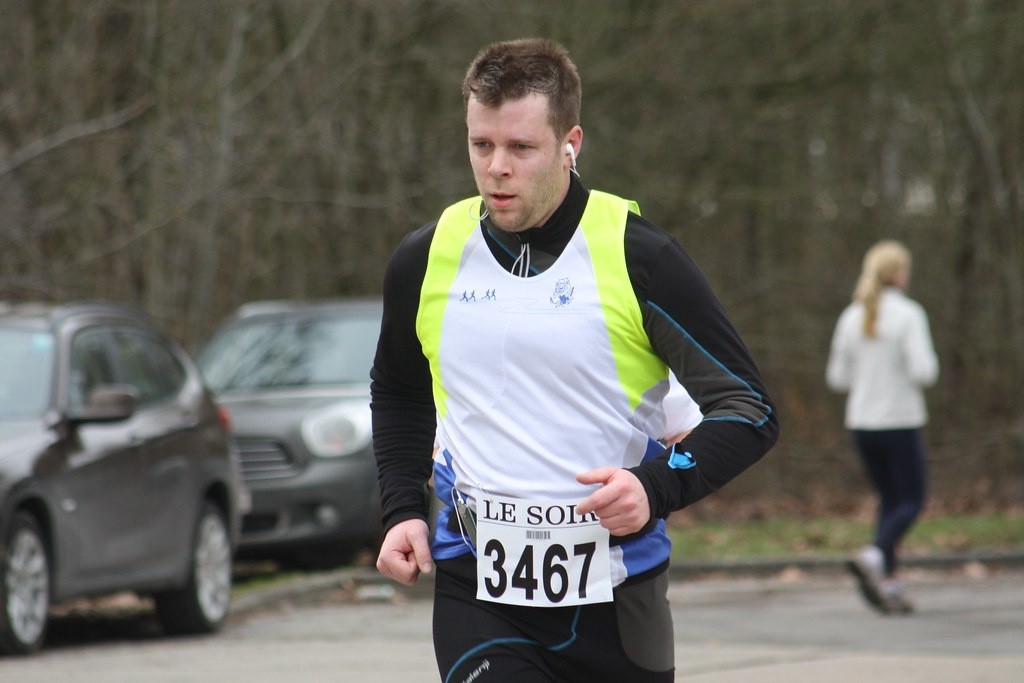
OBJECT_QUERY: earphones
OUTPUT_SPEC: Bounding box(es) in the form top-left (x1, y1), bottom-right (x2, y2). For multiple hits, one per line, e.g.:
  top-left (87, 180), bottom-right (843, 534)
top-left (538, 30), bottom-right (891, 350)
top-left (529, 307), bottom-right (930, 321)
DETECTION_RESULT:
top-left (565, 143), bottom-right (576, 167)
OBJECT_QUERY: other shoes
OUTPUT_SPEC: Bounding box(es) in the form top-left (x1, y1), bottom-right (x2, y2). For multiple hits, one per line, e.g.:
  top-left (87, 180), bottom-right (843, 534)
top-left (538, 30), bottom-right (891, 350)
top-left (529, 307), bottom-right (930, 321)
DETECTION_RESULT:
top-left (847, 549), bottom-right (880, 601)
top-left (874, 593), bottom-right (913, 617)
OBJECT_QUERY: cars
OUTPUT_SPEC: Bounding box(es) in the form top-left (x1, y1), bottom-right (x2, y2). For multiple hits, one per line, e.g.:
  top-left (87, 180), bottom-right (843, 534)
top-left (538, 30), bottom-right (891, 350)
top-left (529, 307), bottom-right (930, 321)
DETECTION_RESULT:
top-left (178, 296), bottom-right (442, 575)
top-left (0, 297), bottom-right (247, 657)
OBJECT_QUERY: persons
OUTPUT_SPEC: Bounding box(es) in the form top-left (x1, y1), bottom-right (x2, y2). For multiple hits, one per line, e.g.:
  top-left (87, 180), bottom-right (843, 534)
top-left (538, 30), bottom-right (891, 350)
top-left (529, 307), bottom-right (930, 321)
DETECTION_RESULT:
top-left (824, 237), bottom-right (939, 615)
top-left (368, 35), bottom-right (780, 683)
